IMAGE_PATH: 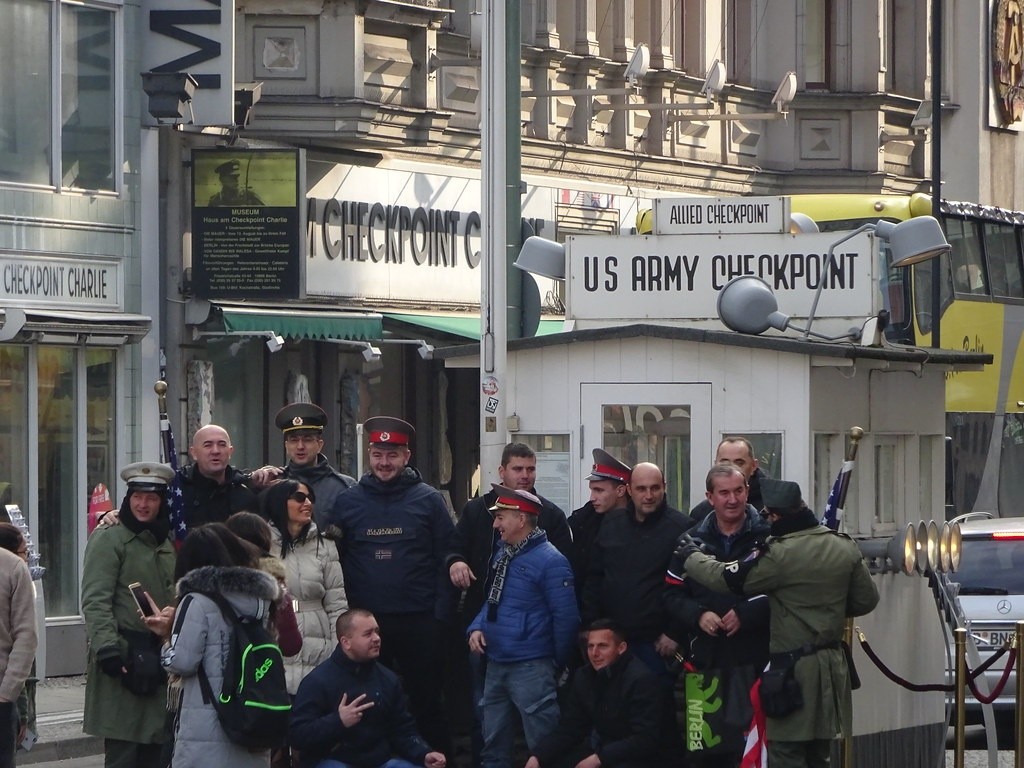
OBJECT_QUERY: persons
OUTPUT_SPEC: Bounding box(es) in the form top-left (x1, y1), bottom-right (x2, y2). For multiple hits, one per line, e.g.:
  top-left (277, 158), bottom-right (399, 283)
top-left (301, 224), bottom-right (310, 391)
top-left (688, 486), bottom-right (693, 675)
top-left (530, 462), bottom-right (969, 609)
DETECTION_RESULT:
top-left (524, 618), bottom-right (683, 768)
top-left (208, 159), bottom-right (266, 205)
top-left (0, 522), bottom-right (39, 750)
top-left (80, 404), bottom-right (768, 768)
top-left (284, 609), bottom-right (446, 768)
top-left (0, 548), bottom-right (39, 768)
top-left (674, 477), bottom-right (881, 768)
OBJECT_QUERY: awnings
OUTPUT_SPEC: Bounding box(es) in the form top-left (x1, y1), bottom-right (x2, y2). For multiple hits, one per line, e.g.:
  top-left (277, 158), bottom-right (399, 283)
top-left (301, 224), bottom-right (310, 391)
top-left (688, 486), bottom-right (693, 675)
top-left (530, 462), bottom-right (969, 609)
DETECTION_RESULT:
top-left (212, 304), bottom-right (384, 342)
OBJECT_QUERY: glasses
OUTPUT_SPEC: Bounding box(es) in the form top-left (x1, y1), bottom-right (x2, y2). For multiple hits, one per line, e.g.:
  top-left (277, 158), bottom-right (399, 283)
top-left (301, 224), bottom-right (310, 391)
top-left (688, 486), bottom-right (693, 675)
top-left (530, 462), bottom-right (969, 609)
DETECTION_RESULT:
top-left (759, 508), bottom-right (782, 520)
top-left (287, 492), bottom-right (315, 504)
top-left (14, 550), bottom-right (27, 555)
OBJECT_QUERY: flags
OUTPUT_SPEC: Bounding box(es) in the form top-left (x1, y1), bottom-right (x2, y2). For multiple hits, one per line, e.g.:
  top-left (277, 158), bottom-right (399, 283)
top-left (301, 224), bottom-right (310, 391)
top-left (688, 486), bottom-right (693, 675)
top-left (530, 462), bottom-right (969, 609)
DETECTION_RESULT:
top-left (168, 421), bottom-right (187, 552)
top-left (741, 468), bottom-right (857, 768)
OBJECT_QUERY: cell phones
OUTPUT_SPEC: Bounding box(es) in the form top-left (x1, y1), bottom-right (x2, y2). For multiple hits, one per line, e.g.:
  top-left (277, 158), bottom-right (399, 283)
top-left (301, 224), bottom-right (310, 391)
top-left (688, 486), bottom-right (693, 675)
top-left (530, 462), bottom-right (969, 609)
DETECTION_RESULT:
top-left (129, 582), bottom-right (155, 621)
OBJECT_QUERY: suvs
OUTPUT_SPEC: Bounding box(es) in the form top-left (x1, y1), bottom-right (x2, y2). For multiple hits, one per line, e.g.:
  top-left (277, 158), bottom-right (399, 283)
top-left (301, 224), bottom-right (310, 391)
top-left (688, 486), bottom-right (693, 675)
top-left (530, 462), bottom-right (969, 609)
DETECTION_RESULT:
top-left (939, 511), bottom-right (1024, 725)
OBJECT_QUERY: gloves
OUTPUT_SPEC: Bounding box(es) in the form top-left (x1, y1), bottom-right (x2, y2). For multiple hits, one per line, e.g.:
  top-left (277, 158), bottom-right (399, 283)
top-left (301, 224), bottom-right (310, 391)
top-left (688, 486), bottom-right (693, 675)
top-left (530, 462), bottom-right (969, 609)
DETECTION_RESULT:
top-left (673, 534), bottom-right (707, 566)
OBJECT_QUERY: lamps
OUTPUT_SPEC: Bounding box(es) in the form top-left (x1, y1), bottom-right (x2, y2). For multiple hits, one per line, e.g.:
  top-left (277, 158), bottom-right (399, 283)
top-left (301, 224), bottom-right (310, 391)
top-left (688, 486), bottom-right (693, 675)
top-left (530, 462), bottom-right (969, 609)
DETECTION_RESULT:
top-left (592, 59), bottom-right (727, 110)
top-left (853, 519), bottom-right (961, 576)
top-left (666, 72), bottom-right (799, 124)
top-left (511, 235), bottom-right (565, 282)
top-left (879, 101), bottom-right (933, 144)
top-left (799, 216), bottom-right (952, 341)
top-left (193, 327), bottom-right (285, 353)
top-left (521, 43), bottom-right (650, 96)
top-left (139, 70), bottom-right (197, 128)
top-left (234, 80), bottom-right (266, 129)
top-left (789, 212), bottom-right (823, 233)
top-left (292, 334), bottom-right (382, 362)
top-left (717, 275), bottom-right (864, 344)
top-left (361, 337), bottom-right (435, 360)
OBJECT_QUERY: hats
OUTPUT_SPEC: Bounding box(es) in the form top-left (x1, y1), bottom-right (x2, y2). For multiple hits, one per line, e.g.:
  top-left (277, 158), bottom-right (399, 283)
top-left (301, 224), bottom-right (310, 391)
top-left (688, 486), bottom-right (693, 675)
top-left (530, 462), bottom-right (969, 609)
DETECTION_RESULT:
top-left (758, 477), bottom-right (803, 509)
top-left (364, 416), bottom-right (415, 449)
top-left (120, 462), bottom-right (175, 492)
top-left (488, 483), bottom-right (543, 517)
top-left (584, 448), bottom-right (632, 487)
top-left (274, 402), bottom-right (328, 440)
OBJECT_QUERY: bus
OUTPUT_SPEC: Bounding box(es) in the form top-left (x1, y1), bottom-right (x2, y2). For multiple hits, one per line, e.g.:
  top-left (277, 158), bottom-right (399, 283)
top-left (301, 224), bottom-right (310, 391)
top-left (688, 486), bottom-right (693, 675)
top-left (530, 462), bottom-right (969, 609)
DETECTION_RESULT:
top-left (631, 191), bottom-right (1024, 519)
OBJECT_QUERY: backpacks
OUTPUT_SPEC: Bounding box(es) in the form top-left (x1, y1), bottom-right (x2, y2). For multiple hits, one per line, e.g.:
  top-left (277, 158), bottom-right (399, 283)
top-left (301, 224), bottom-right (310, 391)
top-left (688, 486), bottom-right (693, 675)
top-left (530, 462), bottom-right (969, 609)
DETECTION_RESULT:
top-left (178, 588), bottom-right (292, 750)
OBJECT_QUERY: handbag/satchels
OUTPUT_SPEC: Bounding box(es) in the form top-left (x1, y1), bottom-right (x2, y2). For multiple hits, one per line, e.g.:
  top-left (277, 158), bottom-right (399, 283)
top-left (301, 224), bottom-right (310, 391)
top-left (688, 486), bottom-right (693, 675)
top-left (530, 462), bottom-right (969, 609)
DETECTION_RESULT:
top-left (672, 628), bottom-right (755, 761)
top-left (759, 665), bottom-right (803, 721)
